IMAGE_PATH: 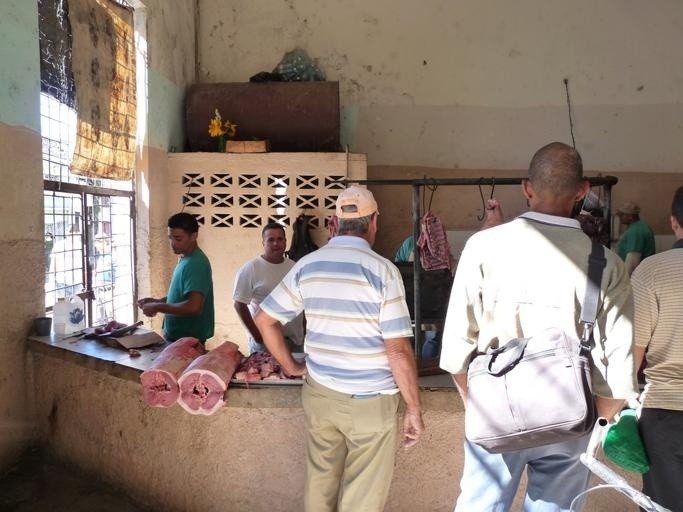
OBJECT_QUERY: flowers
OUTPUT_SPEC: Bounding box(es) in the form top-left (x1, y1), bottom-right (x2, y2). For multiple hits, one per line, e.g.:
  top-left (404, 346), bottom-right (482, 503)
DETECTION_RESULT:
top-left (207, 108), bottom-right (240, 153)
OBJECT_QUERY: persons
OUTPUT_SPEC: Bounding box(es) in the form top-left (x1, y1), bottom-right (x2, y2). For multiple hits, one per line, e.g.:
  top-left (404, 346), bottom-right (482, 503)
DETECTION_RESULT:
top-left (134, 211), bottom-right (216, 352)
top-left (230, 222), bottom-right (303, 354)
top-left (251, 184), bottom-right (425, 511)
top-left (438, 141), bottom-right (640, 512)
top-left (612, 200), bottom-right (656, 276)
top-left (628, 184), bottom-right (682, 510)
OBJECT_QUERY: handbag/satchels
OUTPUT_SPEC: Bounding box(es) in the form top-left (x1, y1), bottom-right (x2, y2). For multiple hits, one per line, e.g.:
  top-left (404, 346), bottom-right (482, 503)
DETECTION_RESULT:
top-left (464, 329), bottom-right (595, 455)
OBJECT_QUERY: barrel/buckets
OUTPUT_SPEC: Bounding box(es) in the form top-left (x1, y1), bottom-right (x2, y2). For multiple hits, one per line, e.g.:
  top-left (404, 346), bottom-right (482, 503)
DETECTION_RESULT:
top-left (65, 294), bottom-right (86, 335)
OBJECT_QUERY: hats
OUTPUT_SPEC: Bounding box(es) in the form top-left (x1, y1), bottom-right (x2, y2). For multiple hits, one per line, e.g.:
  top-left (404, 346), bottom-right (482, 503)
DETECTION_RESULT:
top-left (618, 202), bottom-right (640, 214)
top-left (336, 186), bottom-right (380, 219)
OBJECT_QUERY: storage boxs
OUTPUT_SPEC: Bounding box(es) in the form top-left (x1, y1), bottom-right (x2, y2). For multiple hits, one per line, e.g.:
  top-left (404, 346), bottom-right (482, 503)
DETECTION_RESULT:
top-left (224, 136), bottom-right (270, 152)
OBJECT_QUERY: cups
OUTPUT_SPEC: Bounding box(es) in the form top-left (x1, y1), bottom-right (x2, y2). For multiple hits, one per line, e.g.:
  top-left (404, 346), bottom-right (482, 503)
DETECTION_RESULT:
top-left (33, 315), bottom-right (52, 338)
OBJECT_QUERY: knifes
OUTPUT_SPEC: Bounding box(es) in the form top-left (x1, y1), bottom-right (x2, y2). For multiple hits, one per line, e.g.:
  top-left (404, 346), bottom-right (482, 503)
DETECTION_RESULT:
top-left (93, 320), bottom-right (144, 338)
